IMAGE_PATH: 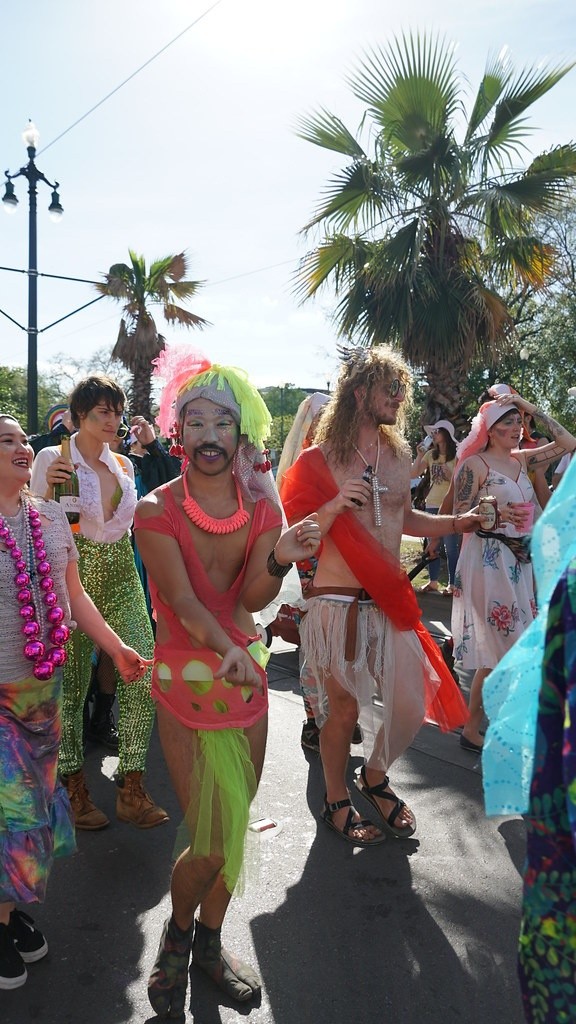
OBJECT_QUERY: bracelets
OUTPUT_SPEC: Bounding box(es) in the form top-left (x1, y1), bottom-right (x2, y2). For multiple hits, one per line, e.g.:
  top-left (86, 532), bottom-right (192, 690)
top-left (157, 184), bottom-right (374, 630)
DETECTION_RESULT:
top-left (266, 550), bottom-right (293, 577)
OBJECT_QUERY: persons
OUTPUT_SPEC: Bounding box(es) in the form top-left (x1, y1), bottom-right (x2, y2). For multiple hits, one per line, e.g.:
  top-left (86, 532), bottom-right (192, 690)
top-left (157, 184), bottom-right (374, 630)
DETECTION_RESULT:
top-left (23, 375), bottom-right (179, 830)
top-left (1, 412), bottom-right (153, 989)
top-left (129, 353), bottom-right (317, 1017)
top-left (276, 348), bottom-right (576, 931)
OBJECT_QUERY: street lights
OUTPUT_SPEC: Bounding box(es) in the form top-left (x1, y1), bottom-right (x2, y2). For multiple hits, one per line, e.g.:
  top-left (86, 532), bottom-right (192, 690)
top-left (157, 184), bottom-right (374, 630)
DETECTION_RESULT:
top-left (325, 377), bottom-right (331, 395)
top-left (0, 117), bottom-right (65, 442)
top-left (278, 381), bottom-right (285, 449)
top-left (518, 348), bottom-right (531, 398)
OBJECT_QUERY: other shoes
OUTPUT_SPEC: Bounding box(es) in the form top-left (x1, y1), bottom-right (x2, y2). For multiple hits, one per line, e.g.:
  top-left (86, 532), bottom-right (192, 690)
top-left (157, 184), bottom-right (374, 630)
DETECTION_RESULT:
top-left (416, 584), bottom-right (440, 595)
top-left (147, 914), bottom-right (195, 1018)
top-left (350, 723), bottom-right (364, 744)
top-left (193, 917), bottom-right (262, 1002)
top-left (443, 590), bottom-right (453, 596)
top-left (301, 722), bottom-right (321, 753)
top-left (460, 734), bottom-right (484, 754)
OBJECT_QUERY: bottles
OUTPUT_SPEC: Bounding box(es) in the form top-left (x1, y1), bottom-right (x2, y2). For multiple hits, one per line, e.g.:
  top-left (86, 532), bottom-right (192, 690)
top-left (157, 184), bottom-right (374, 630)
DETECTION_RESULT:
top-left (54, 433), bottom-right (81, 525)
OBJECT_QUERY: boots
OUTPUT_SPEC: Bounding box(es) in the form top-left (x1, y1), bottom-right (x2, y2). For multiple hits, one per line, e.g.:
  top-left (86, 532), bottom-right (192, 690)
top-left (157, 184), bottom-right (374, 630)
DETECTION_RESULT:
top-left (115, 769), bottom-right (170, 829)
top-left (82, 688), bottom-right (93, 758)
top-left (91, 690), bottom-right (120, 750)
top-left (58, 769), bottom-right (110, 829)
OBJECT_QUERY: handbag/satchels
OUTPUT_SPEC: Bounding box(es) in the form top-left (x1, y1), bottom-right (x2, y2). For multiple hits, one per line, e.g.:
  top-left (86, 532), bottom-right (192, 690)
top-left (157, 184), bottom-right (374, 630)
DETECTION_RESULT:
top-left (475, 529), bottom-right (532, 564)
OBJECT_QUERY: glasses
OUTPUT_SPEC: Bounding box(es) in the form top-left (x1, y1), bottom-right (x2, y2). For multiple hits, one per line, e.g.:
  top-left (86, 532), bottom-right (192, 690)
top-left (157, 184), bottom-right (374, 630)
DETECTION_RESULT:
top-left (116, 422), bottom-right (129, 439)
top-left (383, 377), bottom-right (407, 398)
top-left (431, 429), bottom-right (440, 436)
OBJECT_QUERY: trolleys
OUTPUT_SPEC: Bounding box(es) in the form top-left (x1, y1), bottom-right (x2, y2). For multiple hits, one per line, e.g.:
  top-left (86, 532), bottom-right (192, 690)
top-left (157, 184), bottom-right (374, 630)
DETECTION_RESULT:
top-left (254, 547), bottom-right (464, 692)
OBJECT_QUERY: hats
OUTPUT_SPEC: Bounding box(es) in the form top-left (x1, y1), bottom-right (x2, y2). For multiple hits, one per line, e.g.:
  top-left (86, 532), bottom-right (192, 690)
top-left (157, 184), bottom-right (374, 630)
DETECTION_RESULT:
top-left (423, 420), bottom-right (460, 446)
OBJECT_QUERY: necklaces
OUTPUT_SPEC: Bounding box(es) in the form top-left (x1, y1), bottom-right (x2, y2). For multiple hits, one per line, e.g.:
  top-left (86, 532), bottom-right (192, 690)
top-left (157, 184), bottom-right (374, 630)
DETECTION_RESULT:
top-left (183, 466), bottom-right (251, 533)
top-left (355, 431), bottom-right (389, 526)
top-left (0, 491), bottom-right (71, 681)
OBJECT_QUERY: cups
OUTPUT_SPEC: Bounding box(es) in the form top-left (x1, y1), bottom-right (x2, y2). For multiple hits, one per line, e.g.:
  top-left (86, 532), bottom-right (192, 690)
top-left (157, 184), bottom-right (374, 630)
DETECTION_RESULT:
top-left (418, 435), bottom-right (433, 453)
top-left (512, 502), bottom-right (535, 533)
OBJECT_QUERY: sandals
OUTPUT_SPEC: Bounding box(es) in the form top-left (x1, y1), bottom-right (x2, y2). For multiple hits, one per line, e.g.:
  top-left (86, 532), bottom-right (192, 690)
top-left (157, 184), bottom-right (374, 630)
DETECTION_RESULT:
top-left (355, 763), bottom-right (416, 837)
top-left (320, 792), bottom-right (386, 845)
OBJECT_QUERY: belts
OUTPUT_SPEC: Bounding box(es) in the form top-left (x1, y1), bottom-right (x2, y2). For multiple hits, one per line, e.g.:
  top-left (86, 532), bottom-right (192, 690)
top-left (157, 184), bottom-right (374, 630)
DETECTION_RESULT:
top-left (302, 587), bottom-right (373, 662)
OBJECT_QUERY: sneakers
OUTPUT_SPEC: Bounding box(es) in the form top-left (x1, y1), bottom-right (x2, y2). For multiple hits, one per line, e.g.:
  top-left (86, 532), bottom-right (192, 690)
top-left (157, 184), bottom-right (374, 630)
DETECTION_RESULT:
top-left (438, 638), bottom-right (459, 684)
top-left (6, 908), bottom-right (49, 964)
top-left (0, 923), bottom-right (27, 990)
